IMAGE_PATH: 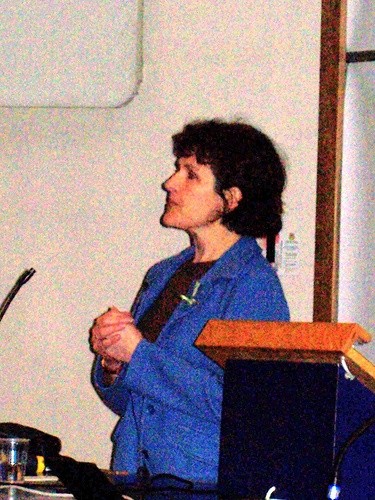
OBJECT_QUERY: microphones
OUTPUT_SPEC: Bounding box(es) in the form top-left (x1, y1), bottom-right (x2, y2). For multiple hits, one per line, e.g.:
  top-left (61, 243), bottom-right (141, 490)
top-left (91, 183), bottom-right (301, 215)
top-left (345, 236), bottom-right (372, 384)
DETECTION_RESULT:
top-left (0, 269), bottom-right (36, 321)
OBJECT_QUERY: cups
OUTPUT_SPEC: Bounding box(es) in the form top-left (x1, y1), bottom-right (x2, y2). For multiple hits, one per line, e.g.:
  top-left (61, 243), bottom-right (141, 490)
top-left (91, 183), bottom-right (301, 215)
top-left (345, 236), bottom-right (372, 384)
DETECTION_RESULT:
top-left (0, 437), bottom-right (31, 484)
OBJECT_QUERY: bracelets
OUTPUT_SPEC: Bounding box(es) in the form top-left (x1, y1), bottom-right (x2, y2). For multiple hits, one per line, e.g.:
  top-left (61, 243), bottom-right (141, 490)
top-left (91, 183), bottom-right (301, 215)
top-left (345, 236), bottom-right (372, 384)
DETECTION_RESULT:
top-left (102, 360), bottom-right (121, 375)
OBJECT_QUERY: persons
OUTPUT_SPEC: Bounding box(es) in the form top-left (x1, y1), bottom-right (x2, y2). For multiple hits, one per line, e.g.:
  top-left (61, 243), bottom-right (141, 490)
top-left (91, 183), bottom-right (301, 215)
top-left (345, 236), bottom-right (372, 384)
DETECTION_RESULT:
top-left (92, 119), bottom-right (290, 500)
